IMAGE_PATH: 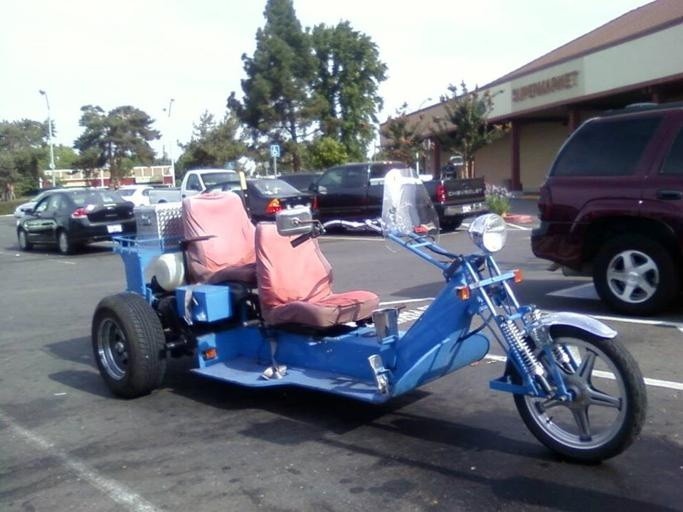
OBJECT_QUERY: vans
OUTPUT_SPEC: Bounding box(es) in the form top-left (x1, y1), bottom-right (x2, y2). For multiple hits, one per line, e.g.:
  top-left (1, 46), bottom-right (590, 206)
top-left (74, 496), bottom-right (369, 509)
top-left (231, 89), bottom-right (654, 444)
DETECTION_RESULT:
top-left (530, 98), bottom-right (683, 317)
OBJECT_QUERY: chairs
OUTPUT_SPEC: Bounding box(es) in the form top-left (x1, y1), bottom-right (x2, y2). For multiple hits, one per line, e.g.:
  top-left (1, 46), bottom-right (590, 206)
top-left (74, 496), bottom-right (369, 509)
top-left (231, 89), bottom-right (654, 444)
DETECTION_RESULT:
top-left (180, 191), bottom-right (379, 330)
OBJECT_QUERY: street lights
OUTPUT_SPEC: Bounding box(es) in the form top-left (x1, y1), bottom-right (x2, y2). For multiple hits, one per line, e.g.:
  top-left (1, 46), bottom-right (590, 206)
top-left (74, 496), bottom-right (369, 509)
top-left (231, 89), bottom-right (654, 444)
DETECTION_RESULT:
top-left (38, 89), bottom-right (56, 190)
top-left (162, 97), bottom-right (176, 187)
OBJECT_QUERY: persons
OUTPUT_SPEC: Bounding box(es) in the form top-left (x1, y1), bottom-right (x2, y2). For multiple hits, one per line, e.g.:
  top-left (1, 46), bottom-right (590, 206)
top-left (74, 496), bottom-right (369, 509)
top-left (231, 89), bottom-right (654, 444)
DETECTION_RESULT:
top-left (442, 161), bottom-right (457, 179)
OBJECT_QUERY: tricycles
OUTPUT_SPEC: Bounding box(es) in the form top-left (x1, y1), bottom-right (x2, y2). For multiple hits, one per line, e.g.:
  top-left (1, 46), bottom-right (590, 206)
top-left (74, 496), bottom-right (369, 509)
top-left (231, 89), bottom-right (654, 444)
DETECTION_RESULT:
top-left (90, 187), bottom-right (649, 467)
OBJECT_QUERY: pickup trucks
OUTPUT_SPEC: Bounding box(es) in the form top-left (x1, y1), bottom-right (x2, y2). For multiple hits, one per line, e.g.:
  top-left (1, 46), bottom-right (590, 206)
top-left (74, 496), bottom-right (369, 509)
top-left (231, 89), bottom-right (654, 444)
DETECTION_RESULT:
top-left (301, 160), bottom-right (490, 231)
